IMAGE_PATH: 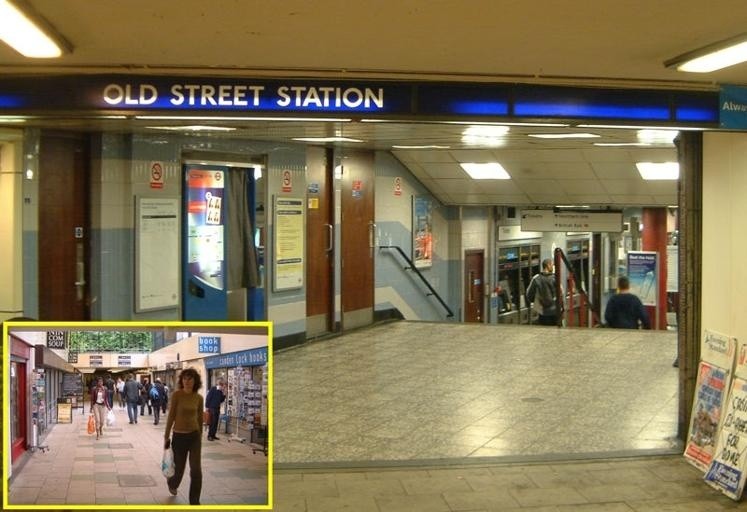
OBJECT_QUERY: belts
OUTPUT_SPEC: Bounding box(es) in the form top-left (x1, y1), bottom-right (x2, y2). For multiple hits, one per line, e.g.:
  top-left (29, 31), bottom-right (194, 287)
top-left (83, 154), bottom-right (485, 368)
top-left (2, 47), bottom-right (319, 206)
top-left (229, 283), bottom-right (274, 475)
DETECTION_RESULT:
top-left (95, 402), bottom-right (104, 405)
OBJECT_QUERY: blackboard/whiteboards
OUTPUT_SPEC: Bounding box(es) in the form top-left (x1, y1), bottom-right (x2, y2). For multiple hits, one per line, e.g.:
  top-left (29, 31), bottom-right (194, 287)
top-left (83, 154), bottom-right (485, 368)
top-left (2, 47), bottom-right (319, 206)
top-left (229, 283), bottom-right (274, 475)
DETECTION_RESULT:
top-left (62, 374), bottom-right (82, 393)
top-left (58, 404), bottom-right (72, 423)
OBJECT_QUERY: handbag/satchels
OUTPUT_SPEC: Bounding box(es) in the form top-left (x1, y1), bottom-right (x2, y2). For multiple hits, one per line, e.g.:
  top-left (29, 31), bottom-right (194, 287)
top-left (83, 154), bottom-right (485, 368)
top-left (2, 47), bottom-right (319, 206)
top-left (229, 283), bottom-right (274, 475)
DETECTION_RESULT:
top-left (87, 414), bottom-right (95, 434)
top-left (204, 412), bottom-right (211, 424)
top-left (150, 388), bottom-right (158, 399)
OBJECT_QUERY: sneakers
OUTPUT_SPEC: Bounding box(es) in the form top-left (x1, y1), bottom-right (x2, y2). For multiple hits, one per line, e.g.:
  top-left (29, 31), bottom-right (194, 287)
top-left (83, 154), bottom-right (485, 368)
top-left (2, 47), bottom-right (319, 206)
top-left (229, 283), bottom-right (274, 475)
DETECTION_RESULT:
top-left (167, 478), bottom-right (178, 496)
top-left (208, 435), bottom-right (220, 441)
top-left (129, 420), bottom-right (137, 424)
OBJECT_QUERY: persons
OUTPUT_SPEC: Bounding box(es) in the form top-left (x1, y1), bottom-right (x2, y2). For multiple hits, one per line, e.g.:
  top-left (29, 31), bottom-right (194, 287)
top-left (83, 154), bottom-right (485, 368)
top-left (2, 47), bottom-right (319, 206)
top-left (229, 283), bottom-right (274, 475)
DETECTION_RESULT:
top-left (163, 369), bottom-right (204, 504)
top-left (525, 259), bottom-right (564, 328)
top-left (205, 381), bottom-right (226, 441)
top-left (87, 373), bottom-right (169, 440)
top-left (605, 277), bottom-right (651, 329)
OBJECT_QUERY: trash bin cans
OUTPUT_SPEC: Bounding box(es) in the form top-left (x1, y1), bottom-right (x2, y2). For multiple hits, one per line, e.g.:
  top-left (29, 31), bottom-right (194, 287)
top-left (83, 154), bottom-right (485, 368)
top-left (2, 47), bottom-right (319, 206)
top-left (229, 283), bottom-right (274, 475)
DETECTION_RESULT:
top-left (39, 419), bottom-right (45, 436)
top-left (30, 418), bottom-right (38, 447)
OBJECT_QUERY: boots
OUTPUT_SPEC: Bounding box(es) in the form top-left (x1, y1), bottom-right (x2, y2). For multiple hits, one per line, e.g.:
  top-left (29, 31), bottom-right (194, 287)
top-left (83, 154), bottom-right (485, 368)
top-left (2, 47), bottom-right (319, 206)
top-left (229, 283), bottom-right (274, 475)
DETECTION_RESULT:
top-left (96, 427), bottom-right (104, 440)
